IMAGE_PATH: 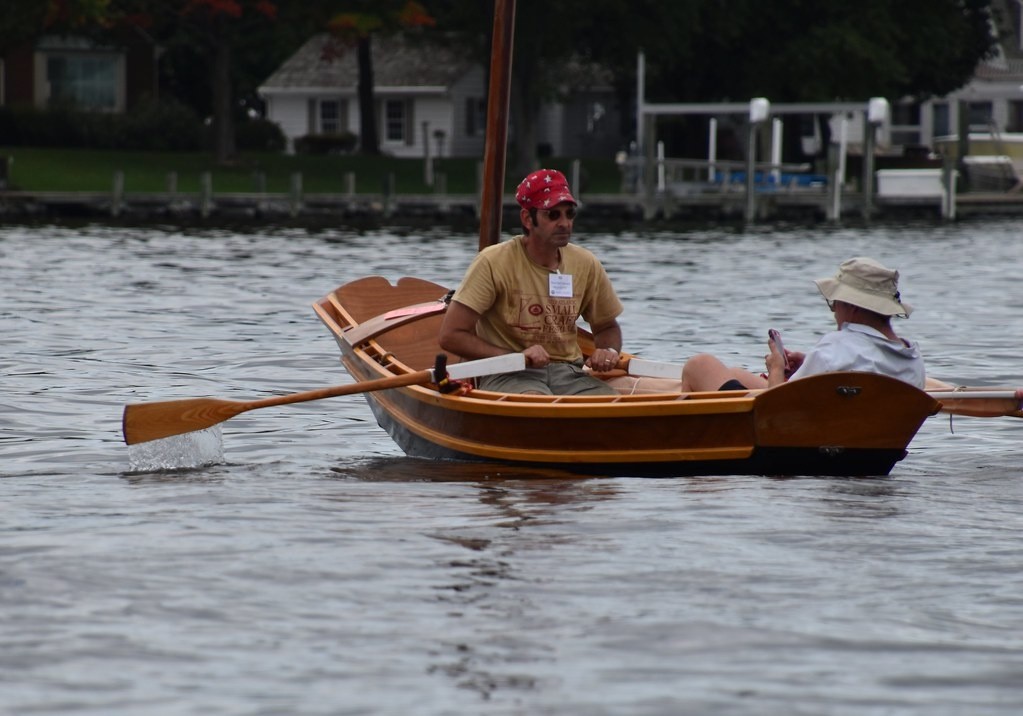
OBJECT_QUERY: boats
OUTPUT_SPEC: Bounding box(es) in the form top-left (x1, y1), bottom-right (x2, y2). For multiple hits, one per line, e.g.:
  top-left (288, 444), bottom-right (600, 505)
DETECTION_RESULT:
top-left (313, 276), bottom-right (944, 480)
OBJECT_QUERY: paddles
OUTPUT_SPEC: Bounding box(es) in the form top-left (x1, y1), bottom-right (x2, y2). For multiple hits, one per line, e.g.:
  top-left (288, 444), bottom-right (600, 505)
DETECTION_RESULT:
top-left (615, 356), bottom-right (1023, 420)
top-left (121, 351), bottom-right (532, 449)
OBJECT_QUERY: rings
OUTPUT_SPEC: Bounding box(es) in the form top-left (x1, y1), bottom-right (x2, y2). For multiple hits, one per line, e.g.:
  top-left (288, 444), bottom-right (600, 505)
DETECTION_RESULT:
top-left (606, 360), bottom-right (610, 362)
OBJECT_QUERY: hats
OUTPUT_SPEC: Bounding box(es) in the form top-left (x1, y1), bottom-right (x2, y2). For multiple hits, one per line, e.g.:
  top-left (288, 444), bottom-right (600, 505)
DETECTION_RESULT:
top-left (813, 256), bottom-right (913, 319)
top-left (515, 169), bottom-right (578, 210)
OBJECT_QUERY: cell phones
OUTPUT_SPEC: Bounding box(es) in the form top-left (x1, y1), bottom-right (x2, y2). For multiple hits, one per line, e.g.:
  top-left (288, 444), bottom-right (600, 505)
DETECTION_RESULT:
top-left (768, 329), bottom-right (790, 370)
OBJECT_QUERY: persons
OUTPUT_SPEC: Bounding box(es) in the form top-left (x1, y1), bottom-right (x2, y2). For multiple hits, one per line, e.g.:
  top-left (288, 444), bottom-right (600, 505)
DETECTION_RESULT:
top-left (681, 256), bottom-right (926, 392)
top-left (438, 168), bottom-right (624, 396)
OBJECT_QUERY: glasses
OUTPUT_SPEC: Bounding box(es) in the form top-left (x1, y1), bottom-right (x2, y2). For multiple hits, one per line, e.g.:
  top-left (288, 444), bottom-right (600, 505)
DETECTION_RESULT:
top-left (537, 209), bottom-right (577, 221)
top-left (826, 298), bottom-right (835, 312)
